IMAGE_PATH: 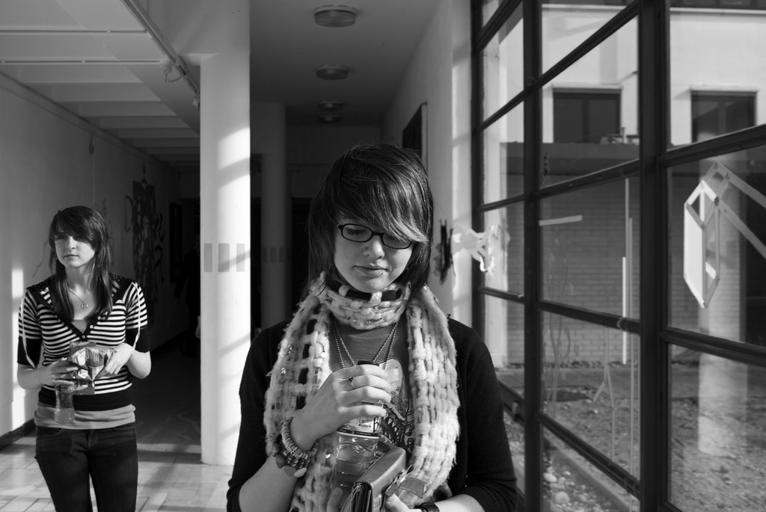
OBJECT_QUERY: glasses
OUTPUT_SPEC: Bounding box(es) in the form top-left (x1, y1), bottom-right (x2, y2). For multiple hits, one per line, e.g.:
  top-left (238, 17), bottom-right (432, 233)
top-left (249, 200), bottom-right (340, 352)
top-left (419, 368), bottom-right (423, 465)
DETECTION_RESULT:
top-left (336, 223), bottom-right (412, 248)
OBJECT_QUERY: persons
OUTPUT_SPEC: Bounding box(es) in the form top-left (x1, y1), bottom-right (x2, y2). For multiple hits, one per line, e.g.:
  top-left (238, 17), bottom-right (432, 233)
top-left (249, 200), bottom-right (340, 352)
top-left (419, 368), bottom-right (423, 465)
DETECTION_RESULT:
top-left (17, 206), bottom-right (153, 512)
top-left (226, 143), bottom-right (518, 512)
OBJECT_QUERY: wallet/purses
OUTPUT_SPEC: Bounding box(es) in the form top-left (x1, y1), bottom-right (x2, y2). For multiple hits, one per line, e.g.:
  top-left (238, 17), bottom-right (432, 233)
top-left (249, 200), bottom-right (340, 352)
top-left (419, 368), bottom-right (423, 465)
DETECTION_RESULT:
top-left (341, 448), bottom-right (406, 512)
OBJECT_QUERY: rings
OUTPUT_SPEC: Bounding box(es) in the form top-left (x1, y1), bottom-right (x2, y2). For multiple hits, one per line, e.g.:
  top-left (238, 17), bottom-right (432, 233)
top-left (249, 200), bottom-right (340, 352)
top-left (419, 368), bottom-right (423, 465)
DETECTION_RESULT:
top-left (349, 377), bottom-right (356, 392)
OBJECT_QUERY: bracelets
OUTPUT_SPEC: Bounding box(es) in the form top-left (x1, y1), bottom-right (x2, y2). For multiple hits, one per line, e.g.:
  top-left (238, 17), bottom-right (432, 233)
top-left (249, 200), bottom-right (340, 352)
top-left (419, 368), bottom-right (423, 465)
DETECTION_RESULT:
top-left (415, 502), bottom-right (439, 512)
top-left (274, 417), bottom-right (320, 478)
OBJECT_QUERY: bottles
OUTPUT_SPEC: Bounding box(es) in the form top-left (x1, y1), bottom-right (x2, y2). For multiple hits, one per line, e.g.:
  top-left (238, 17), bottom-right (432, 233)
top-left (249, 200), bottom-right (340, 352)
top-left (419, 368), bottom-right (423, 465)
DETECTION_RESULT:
top-left (329, 359), bottom-right (384, 510)
top-left (53, 355), bottom-right (76, 426)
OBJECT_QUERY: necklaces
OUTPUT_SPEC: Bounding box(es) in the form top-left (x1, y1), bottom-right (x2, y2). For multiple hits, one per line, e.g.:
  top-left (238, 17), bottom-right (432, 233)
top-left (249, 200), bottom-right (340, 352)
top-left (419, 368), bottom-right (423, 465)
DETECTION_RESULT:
top-left (331, 321), bottom-right (398, 369)
top-left (70, 288), bottom-right (93, 308)
